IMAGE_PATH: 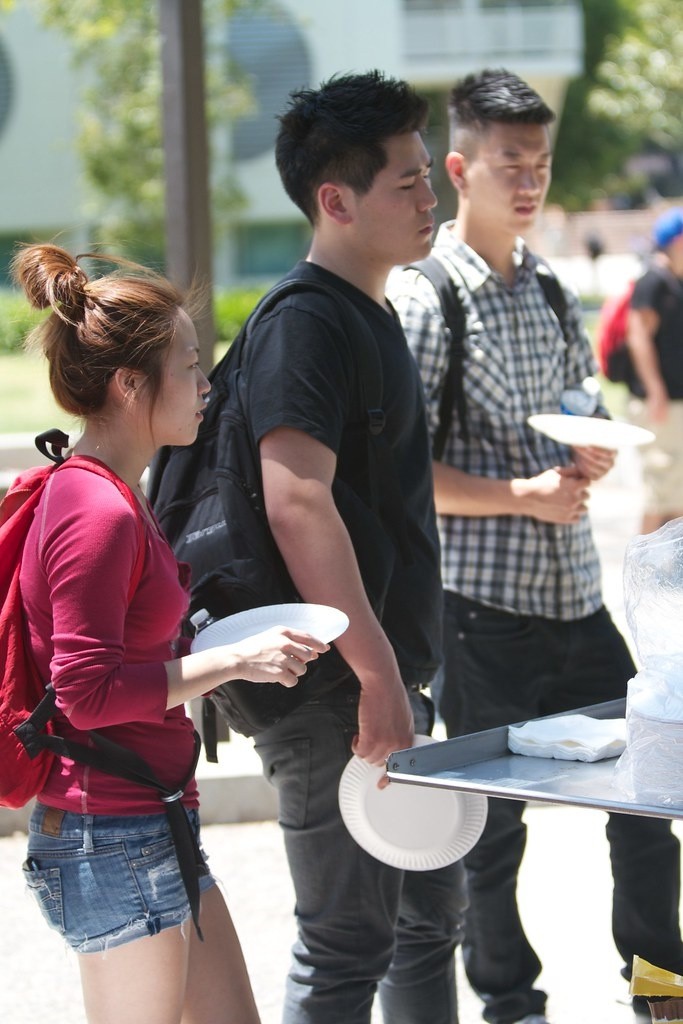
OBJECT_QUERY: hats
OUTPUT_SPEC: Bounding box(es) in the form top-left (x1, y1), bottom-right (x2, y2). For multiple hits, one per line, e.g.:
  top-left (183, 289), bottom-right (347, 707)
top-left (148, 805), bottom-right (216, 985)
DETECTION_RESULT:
top-left (652, 206), bottom-right (683, 250)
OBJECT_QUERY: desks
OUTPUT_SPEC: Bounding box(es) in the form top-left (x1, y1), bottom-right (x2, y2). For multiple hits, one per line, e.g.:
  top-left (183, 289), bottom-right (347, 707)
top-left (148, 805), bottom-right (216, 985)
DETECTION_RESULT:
top-left (386, 694), bottom-right (683, 821)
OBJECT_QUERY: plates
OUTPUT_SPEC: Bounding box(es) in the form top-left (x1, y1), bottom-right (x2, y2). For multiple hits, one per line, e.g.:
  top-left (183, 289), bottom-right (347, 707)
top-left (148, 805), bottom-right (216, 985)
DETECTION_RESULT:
top-left (338, 734), bottom-right (489, 872)
top-left (527, 413), bottom-right (658, 450)
top-left (189, 602), bottom-right (349, 663)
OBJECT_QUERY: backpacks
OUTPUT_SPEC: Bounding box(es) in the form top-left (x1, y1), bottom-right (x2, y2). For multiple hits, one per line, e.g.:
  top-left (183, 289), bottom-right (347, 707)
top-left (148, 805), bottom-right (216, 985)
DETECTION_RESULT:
top-left (0, 429), bottom-right (149, 810)
top-left (596, 267), bottom-right (673, 383)
top-left (147, 275), bottom-right (362, 740)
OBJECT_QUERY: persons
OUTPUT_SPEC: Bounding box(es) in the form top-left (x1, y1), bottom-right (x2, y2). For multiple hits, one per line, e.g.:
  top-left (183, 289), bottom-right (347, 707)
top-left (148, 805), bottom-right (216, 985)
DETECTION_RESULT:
top-left (389, 72), bottom-right (683, 1022)
top-left (24, 242), bottom-right (329, 1024)
top-left (250, 71), bottom-right (468, 1023)
top-left (626, 208), bottom-right (683, 533)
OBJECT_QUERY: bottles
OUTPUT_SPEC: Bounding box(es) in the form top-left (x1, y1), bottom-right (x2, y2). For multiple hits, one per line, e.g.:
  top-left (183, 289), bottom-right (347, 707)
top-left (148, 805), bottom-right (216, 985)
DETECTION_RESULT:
top-left (189, 608), bottom-right (221, 638)
top-left (561, 376), bottom-right (601, 416)
